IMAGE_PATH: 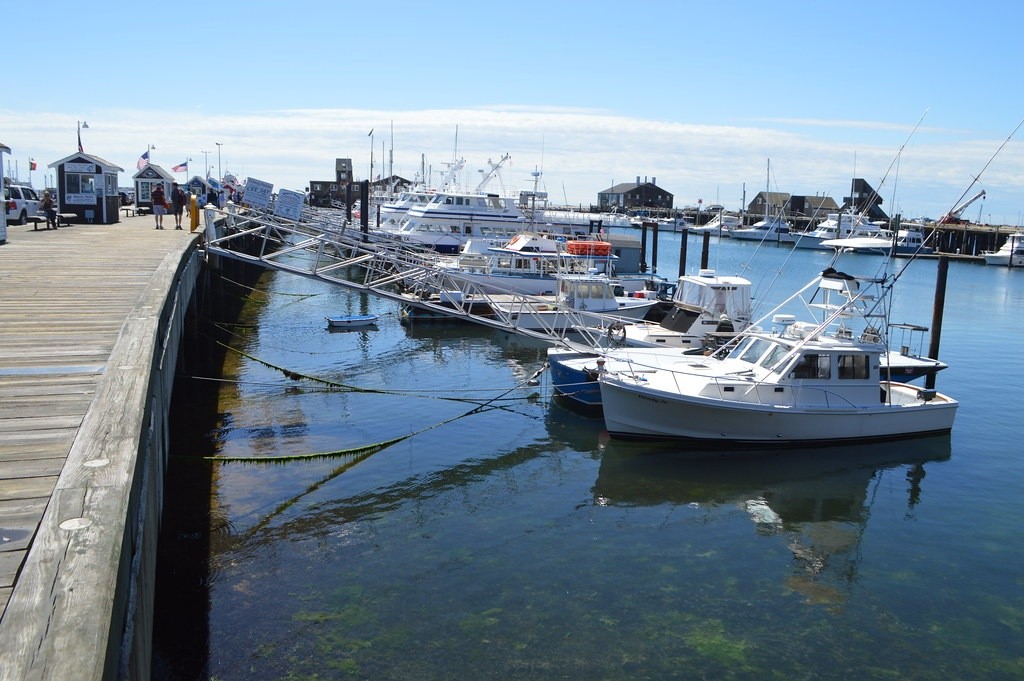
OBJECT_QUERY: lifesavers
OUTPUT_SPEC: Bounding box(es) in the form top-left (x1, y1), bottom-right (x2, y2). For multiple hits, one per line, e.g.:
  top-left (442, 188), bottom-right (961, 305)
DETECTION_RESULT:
top-left (487, 193), bottom-right (499, 198)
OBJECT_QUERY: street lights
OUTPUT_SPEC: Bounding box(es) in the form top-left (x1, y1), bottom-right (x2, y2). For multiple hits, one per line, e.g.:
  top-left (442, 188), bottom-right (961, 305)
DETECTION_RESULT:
top-left (216, 142), bottom-right (223, 182)
top-left (201, 150), bottom-right (211, 180)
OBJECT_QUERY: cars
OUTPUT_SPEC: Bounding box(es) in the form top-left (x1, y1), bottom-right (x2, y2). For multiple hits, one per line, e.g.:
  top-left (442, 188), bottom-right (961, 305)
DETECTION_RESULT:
top-left (4, 184), bottom-right (42, 226)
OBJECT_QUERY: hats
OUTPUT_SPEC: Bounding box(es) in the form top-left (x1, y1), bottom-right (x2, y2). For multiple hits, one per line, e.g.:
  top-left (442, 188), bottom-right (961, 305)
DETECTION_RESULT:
top-left (157, 183), bottom-right (164, 186)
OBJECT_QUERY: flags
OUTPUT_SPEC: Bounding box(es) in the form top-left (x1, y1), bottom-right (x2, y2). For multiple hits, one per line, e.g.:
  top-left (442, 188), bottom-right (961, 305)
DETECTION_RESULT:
top-left (172, 162), bottom-right (187, 172)
top-left (78, 135), bottom-right (84, 153)
top-left (137, 151), bottom-right (148, 171)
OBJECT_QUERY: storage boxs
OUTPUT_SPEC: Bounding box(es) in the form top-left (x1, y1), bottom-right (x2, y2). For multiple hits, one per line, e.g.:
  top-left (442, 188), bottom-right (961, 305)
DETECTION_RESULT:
top-left (634, 292), bottom-right (643, 298)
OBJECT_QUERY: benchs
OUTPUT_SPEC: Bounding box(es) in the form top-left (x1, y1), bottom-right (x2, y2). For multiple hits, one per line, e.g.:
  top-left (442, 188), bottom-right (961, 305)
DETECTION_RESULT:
top-left (138, 207), bottom-right (150, 215)
top-left (25, 215), bottom-right (58, 230)
top-left (56, 214), bottom-right (78, 227)
top-left (122, 208), bottom-right (139, 217)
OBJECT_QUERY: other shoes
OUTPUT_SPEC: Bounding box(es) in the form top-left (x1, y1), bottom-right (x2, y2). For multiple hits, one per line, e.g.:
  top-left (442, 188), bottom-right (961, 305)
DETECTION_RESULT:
top-left (156, 226), bottom-right (163, 229)
top-left (176, 226), bottom-right (182, 230)
top-left (52, 222), bottom-right (57, 229)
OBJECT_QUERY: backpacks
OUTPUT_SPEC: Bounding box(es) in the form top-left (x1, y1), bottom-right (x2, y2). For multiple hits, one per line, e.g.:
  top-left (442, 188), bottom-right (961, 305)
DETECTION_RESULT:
top-left (178, 189), bottom-right (187, 205)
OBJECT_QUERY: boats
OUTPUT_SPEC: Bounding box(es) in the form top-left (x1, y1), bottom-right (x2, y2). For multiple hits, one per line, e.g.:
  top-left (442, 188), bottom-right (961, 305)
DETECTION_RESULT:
top-left (324, 313), bottom-right (380, 327)
top-left (978, 229), bottom-right (1024, 268)
top-left (334, 106), bottom-right (1023, 444)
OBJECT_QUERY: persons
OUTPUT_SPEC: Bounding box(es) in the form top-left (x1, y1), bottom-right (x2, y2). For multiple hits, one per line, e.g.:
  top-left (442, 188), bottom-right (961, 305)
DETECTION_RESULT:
top-left (151, 183), bottom-right (165, 229)
top-left (207, 188), bottom-right (217, 207)
top-left (228, 191), bottom-right (244, 204)
top-left (215, 191), bottom-right (227, 210)
top-left (184, 188), bottom-right (193, 218)
top-left (41, 191), bottom-right (57, 229)
top-left (171, 182), bottom-right (183, 230)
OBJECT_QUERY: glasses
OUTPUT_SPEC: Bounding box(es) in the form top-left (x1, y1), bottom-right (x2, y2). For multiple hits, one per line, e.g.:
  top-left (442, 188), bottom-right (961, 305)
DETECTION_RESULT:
top-left (44, 195), bottom-right (49, 196)
